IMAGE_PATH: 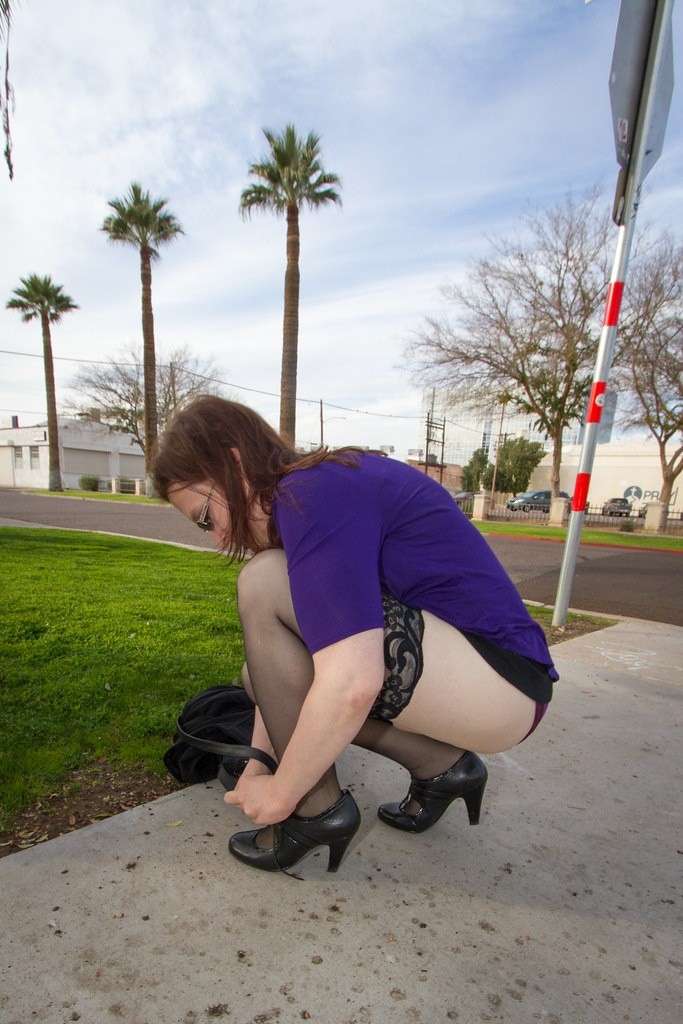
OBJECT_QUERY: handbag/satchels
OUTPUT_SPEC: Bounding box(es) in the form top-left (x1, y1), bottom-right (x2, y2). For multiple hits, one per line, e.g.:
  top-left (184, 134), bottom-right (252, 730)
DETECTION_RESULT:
top-left (163, 685), bottom-right (278, 792)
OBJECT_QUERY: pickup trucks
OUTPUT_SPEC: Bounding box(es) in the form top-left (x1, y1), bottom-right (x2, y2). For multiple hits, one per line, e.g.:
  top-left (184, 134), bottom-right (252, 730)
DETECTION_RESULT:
top-left (570, 497), bottom-right (590, 514)
top-left (602, 499), bottom-right (633, 517)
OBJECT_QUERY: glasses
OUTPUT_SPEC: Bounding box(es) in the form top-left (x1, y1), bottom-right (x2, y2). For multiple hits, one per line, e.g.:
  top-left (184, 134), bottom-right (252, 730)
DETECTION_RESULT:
top-left (196, 487), bottom-right (215, 532)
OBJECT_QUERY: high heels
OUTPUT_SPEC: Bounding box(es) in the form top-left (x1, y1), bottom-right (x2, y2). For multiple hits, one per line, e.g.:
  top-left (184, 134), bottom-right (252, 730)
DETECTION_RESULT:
top-left (377, 752), bottom-right (488, 833)
top-left (229, 789), bottom-right (361, 873)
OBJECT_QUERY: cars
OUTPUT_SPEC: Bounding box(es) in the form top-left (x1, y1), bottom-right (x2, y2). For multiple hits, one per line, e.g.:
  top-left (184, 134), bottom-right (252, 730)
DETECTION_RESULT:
top-left (639, 504), bottom-right (648, 519)
top-left (507, 490), bottom-right (570, 513)
top-left (453, 492), bottom-right (481, 503)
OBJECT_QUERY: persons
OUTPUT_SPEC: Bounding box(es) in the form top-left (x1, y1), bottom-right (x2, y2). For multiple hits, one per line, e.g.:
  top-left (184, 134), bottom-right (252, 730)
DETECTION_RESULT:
top-left (151, 391), bottom-right (557, 881)
